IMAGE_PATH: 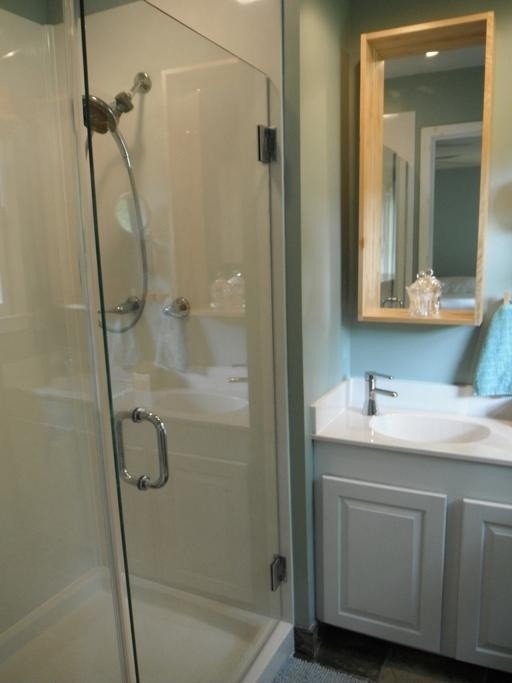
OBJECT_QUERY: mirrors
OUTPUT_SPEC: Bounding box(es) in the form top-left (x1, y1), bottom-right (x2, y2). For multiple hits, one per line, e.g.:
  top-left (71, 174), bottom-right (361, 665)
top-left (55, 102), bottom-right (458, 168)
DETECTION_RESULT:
top-left (356, 9), bottom-right (495, 329)
top-left (418, 118), bottom-right (484, 305)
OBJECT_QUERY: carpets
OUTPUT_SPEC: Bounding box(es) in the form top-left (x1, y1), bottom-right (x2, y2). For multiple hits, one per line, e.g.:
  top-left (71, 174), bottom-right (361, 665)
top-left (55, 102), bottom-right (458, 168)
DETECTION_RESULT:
top-left (274, 655), bottom-right (374, 683)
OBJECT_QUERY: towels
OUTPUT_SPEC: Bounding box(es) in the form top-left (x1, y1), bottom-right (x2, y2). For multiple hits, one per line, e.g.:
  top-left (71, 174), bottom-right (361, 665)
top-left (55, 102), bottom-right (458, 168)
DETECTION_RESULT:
top-left (472, 295), bottom-right (512, 397)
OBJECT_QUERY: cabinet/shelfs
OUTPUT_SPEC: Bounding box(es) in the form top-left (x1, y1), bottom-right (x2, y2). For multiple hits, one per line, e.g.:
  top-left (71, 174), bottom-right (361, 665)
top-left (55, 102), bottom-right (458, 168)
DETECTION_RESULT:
top-left (310, 440), bottom-right (511, 675)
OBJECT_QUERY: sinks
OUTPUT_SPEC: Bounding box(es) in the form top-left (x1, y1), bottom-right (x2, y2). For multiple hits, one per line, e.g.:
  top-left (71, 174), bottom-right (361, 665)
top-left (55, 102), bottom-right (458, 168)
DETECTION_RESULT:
top-left (139, 387), bottom-right (249, 418)
top-left (364, 411), bottom-right (493, 448)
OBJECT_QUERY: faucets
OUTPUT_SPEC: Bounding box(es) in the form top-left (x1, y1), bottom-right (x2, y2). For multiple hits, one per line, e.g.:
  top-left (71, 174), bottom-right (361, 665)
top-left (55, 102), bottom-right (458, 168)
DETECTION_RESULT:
top-left (381, 295), bottom-right (404, 308)
top-left (225, 374), bottom-right (249, 386)
top-left (365, 370), bottom-right (400, 415)
